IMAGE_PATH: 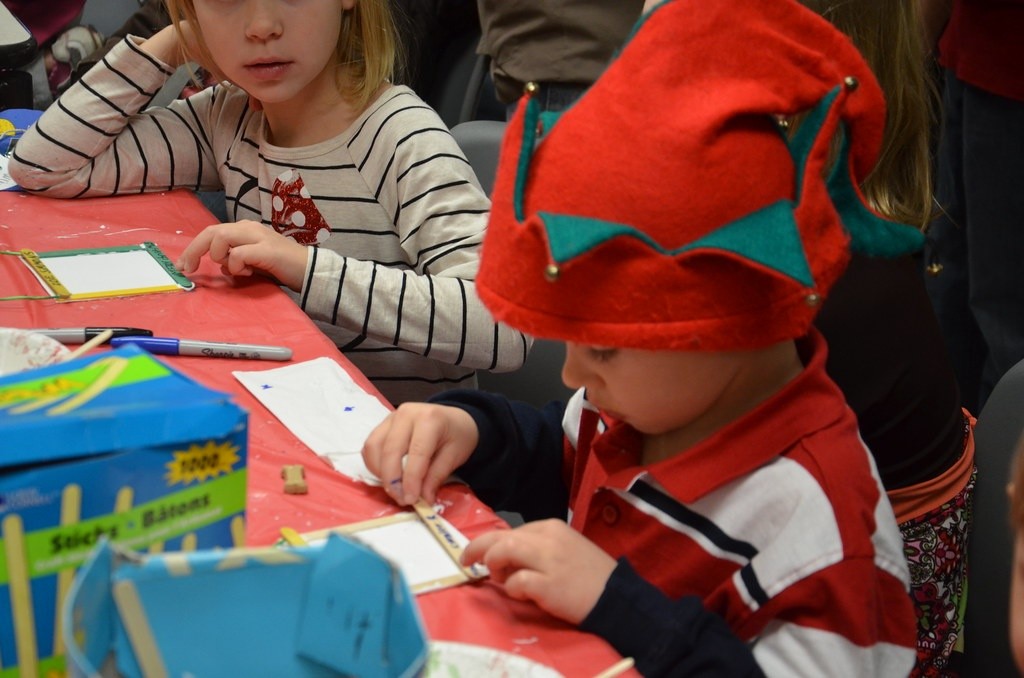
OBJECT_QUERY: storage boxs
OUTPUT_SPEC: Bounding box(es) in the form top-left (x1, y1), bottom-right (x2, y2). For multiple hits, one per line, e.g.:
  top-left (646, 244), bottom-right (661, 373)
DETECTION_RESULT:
top-left (0, 342), bottom-right (250, 678)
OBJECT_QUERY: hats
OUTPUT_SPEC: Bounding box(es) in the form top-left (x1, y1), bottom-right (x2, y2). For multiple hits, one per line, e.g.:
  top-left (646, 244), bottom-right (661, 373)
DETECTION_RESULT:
top-left (476, 1), bottom-right (924, 351)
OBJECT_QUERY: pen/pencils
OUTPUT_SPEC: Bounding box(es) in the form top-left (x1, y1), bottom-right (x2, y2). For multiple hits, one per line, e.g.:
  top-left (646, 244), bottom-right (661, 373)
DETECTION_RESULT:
top-left (29, 327), bottom-right (154, 343)
top-left (111, 336), bottom-right (292, 361)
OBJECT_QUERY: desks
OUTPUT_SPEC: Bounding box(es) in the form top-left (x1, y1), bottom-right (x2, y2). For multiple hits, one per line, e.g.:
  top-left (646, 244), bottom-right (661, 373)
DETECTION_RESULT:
top-left (0, 188), bottom-right (641, 678)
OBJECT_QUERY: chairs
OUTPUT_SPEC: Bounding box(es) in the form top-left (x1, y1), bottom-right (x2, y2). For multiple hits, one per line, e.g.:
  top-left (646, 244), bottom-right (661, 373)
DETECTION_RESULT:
top-left (451, 119), bottom-right (576, 407)
top-left (955, 360), bottom-right (1024, 678)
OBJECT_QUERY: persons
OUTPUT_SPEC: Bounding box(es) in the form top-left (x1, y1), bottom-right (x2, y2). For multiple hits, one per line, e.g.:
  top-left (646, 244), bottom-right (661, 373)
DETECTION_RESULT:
top-left (793, 0), bottom-right (976, 678)
top-left (6, 0), bottom-right (534, 411)
top-left (360, 0), bottom-right (919, 678)
top-left (0, 0), bottom-right (1023, 411)
